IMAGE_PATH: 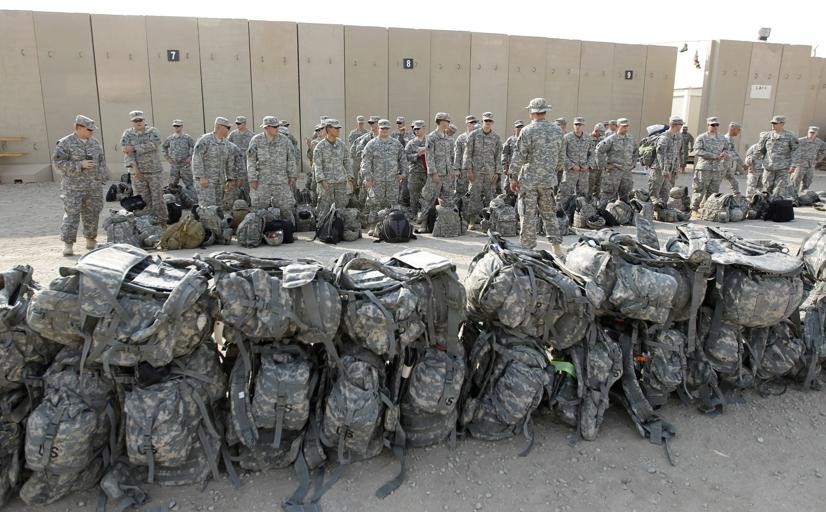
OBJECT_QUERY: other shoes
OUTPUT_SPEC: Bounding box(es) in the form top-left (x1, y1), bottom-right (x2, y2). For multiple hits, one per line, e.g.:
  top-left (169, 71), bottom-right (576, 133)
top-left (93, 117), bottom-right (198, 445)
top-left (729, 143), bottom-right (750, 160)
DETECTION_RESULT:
top-left (468, 223), bottom-right (476, 230)
top-left (63, 244), bottom-right (74, 256)
top-left (85, 237), bottom-right (97, 249)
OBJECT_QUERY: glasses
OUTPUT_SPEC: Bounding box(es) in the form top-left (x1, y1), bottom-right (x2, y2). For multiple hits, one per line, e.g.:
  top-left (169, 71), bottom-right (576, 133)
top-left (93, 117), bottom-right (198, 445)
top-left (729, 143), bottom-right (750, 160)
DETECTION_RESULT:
top-left (711, 124), bottom-right (719, 127)
top-left (133, 119), bottom-right (142, 122)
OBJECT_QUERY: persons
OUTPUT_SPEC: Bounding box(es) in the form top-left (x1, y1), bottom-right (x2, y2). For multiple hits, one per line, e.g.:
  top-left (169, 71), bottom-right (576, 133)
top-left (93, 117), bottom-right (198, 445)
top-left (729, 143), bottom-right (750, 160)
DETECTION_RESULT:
top-left (52, 114), bottom-right (106, 255)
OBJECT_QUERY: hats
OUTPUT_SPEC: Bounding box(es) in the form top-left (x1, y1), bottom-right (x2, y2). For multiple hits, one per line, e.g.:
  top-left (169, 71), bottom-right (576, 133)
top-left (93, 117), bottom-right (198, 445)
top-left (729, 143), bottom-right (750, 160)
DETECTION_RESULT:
top-left (553, 117), bottom-right (629, 136)
top-left (130, 112), bottom-right (144, 120)
top-left (171, 120), bottom-right (184, 125)
top-left (808, 127), bottom-right (819, 132)
top-left (435, 112), bottom-right (494, 132)
top-left (316, 115), bottom-right (426, 131)
top-left (769, 117), bottom-right (785, 123)
top-left (730, 123), bottom-right (743, 130)
top-left (514, 120), bottom-right (523, 126)
top-left (706, 116), bottom-right (719, 124)
top-left (76, 114), bottom-right (98, 130)
top-left (215, 116), bottom-right (290, 128)
top-left (670, 118), bottom-right (688, 130)
top-left (524, 98), bottom-right (552, 113)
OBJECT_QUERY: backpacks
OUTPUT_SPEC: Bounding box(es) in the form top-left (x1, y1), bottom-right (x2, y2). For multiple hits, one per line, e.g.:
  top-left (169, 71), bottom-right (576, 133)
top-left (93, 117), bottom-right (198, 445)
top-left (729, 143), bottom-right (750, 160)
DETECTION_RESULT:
top-left (639, 124), bottom-right (669, 166)
top-left (313, 200), bottom-right (466, 242)
top-left (0, 243), bottom-right (480, 506)
top-left (635, 184), bottom-right (826, 221)
top-left (104, 206), bottom-right (294, 250)
top-left (466, 225), bottom-right (826, 441)
top-left (481, 192), bottom-right (635, 237)
